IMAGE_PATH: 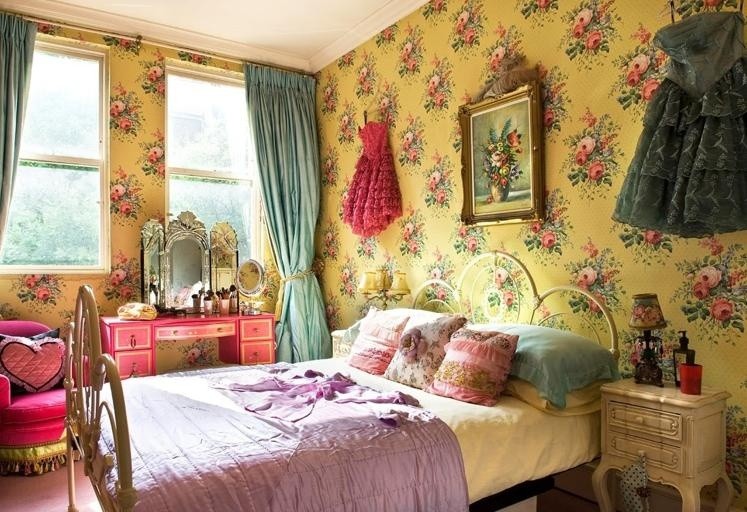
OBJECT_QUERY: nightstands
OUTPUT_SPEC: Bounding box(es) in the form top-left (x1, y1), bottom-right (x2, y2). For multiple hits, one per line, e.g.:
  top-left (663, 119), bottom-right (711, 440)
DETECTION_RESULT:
top-left (589, 376), bottom-right (733, 511)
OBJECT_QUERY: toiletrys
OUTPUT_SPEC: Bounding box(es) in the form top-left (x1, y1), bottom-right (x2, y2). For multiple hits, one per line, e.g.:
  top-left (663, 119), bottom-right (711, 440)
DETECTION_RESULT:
top-left (239, 300), bottom-right (263, 316)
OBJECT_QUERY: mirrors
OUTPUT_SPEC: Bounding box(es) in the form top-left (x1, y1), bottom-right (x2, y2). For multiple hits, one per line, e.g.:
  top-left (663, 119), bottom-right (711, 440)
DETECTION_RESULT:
top-left (139, 212), bottom-right (240, 314)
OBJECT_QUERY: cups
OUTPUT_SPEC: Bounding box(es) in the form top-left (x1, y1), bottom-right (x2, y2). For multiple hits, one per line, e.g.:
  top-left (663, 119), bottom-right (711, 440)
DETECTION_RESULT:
top-left (204, 299), bottom-right (212, 314)
top-left (219, 298), bottom-right (230, 315)
top-left (193, 297), bottom-right (200, 306)
top-left (679, 363), bottom-right (703, 396)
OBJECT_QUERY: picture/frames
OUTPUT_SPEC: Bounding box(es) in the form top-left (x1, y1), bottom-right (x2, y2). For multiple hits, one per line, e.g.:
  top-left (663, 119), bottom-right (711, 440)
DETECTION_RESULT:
top-left (456, 78), bottom-right (545, 227)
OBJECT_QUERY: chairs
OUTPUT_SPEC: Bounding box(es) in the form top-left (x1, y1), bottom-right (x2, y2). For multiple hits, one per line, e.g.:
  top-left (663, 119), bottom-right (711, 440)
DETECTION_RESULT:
top-left (0, 321), bottom-right (91, 475)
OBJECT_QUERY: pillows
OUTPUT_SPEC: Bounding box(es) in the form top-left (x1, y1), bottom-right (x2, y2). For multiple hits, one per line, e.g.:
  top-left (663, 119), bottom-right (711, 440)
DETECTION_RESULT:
top-left (343, 306), bottom-right (613, 417)
top-left (0, 328), bottom-right (64, 394)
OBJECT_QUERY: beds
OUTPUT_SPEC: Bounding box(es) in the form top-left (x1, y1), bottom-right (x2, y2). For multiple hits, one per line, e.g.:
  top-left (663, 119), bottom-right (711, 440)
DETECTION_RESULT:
top-left (64, 250), bottom-right (618, 511)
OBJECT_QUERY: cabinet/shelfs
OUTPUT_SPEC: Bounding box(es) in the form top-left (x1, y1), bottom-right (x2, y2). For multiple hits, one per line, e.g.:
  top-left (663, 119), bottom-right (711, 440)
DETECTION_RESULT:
top-left (98, 311), bottom-right (275, 380)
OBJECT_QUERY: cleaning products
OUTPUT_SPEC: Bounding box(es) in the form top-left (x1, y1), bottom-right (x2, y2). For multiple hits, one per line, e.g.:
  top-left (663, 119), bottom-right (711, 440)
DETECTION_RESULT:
top-left (680, 349), bottom-right (702, 395)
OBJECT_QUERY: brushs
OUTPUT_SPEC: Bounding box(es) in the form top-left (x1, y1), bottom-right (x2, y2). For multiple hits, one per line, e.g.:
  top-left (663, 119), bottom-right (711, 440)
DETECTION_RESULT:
top-left (191, 289), bottom-right (204, 299)
top-left (202, 284), bottom-right (237, 300)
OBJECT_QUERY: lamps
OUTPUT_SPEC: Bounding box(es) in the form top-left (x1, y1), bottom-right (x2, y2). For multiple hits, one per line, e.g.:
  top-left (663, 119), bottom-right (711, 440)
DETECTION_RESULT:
top-left (356, 269), bottom-right (410, 309)
top-left (628, 294), bottom-right (666, 388)
